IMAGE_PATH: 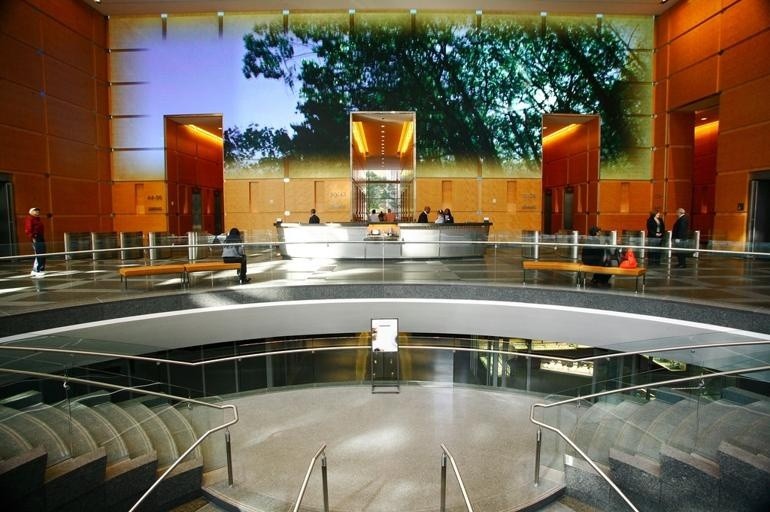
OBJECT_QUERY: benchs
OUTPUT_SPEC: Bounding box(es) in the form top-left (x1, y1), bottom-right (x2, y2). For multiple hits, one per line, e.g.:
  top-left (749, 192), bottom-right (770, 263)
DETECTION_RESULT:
top-left (119, 262), bottom-right (242, 291)
top-left (521, 260), bottom-right (647, 297)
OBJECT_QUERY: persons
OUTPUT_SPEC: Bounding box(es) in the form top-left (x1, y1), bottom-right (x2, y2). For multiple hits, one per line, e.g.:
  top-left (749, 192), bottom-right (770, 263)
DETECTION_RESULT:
top-left (369, 210), bottom-right (380, 222)
top-left (434, 210), bottom-right (444, 223)
top-left (418, 206), bottom-right (431, 222)
top-left (582, 226), bottom-right (613, 289)
top-left (671, 208), bottom-right (689, 268)
top-left (309, 209), bottom-right (320, 223)
top-left (444, 208), bottom-right (454, 223)
top-left (647, 209), bottom-right (665, 267)
top-left (384, 209), bottom-right (397, 222)
top-left (26, 207), bottom-right (47, 276)
top-left (378, 211), bottom-right (385, 221)
top-left (222, 228), bottom-right (252, 283)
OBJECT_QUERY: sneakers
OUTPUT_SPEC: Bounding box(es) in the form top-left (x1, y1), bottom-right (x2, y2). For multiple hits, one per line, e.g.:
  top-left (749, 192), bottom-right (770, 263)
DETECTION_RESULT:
top-left (31, 269), bottom-right (46, 276)
top-left (239, 275), bottom-right (251, 283)
